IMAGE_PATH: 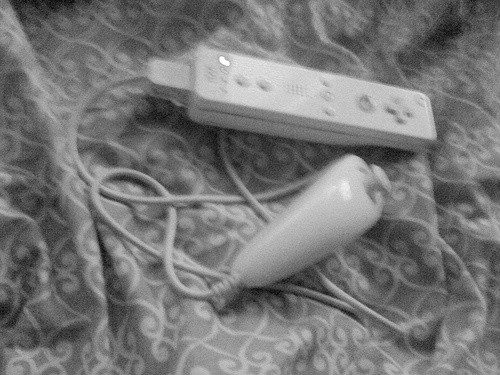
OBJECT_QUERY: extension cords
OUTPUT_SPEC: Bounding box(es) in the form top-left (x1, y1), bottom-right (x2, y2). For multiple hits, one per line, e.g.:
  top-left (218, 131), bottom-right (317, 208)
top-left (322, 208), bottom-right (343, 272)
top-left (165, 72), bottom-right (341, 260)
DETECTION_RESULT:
top-left (188, 49), bottom-right (438, 155)
